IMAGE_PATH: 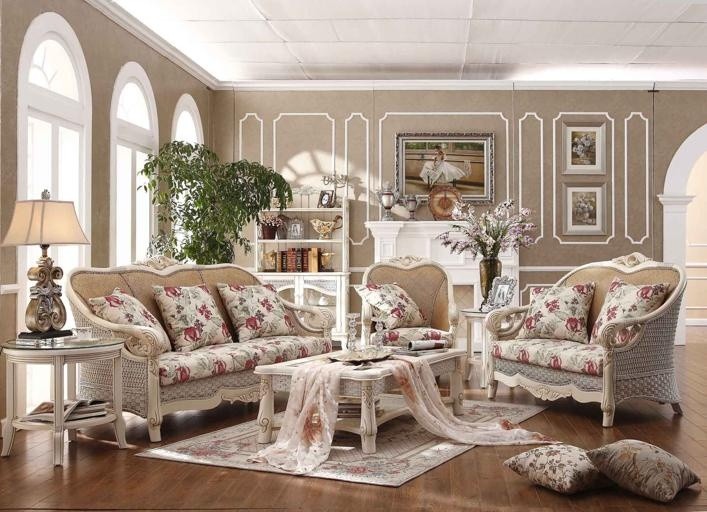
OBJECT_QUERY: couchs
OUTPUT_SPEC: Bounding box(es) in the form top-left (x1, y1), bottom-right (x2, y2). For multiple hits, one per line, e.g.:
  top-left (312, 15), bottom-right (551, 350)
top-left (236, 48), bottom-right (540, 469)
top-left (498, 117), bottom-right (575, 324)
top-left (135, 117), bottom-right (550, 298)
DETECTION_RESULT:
top-left (65, 262), bottom-right (337, 444)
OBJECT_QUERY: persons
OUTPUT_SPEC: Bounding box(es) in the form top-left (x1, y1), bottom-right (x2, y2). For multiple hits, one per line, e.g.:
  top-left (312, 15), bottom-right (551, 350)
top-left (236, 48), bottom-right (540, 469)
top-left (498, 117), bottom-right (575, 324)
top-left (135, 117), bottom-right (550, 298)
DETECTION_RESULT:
top-left (421, 145), bottom-right (465, 190)
top-left (292, 224), bottom-right (299, 237)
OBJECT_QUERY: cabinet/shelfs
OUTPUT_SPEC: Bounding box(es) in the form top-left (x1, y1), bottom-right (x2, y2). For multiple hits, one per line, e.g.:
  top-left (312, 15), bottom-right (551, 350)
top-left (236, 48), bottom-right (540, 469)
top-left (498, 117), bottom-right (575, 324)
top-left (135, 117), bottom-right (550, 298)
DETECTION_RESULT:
top-left (251, 196), bottom-right (350, 349)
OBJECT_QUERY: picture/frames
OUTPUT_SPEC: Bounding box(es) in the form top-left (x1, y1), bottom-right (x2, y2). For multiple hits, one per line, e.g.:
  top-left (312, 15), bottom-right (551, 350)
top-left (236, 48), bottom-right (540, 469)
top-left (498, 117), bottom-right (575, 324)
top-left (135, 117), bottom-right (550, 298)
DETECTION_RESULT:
top-left (487, 275), bottom-right (517, 313)
top-left (562, 181), bottom-right (608, 237)
top-left (562, 121), bottom-right (607, 177)
top-left (395, 131), bottom-right (495, 206)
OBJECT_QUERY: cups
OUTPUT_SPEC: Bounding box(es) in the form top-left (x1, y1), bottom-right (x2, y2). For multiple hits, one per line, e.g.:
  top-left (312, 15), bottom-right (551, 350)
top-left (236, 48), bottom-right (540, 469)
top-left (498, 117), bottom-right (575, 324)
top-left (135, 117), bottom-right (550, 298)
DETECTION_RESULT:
top-left (72, 326), bottom-right (95, 340)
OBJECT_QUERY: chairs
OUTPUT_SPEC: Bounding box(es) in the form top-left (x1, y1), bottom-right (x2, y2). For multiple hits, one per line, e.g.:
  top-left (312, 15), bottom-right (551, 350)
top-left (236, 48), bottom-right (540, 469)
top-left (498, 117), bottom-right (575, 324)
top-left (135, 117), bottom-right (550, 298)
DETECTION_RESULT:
top-left (360, 255), bottom-right (459, 352)
top-left (485, 253), bottom-right (687, 430)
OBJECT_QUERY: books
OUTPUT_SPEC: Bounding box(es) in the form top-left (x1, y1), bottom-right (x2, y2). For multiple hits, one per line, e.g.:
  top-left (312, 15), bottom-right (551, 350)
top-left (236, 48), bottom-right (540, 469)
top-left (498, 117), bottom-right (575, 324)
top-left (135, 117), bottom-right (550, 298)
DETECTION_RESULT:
top-left (276, 247), bottom-right (321, 272)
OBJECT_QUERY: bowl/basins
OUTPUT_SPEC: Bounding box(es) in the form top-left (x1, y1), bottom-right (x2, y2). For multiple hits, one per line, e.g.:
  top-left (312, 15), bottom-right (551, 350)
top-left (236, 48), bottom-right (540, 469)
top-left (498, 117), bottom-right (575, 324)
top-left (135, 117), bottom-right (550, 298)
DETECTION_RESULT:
top-left (273, 197), bottom-right (292, 208)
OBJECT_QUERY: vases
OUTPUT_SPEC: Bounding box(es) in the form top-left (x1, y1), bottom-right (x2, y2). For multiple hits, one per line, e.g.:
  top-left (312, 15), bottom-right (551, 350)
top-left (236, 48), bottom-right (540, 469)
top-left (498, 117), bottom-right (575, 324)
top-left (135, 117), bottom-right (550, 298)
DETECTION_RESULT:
top-left (478, 252), bottom-right (502, 312)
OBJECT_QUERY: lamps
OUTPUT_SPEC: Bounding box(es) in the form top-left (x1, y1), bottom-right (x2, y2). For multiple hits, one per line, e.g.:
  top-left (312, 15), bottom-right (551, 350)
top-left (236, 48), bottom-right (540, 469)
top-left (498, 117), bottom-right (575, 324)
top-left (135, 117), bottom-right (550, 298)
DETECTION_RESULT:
top-left (0, 189), bottom-right (90, 339)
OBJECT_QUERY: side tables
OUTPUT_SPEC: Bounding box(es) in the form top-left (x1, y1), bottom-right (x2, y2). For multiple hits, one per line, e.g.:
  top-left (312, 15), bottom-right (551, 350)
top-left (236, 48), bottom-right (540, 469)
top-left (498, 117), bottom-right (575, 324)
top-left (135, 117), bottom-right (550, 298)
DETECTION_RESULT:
top-left (1, 337), bottom-right (127, 465)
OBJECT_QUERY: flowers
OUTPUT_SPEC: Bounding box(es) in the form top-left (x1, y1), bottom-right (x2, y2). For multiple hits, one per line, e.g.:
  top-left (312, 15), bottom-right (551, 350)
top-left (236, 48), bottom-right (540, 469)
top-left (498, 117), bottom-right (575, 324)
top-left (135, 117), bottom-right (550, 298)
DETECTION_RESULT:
top-left (436, 198), bottom-right (538, 261)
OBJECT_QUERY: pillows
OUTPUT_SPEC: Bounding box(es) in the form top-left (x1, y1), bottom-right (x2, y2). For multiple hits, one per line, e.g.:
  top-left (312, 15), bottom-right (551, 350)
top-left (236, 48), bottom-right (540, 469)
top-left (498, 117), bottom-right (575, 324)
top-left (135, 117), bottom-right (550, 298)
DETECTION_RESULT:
top-left (501, 443), bottom-right (617, 496)
top-left (89, 286), bottom-right (172, 356)
top-left (586, 439), bottom-right (702, 504)
top-left (353, 283), bottom-right (428, 332)
top-left (589, 277), bottom-right (670, 347)
top-left (515, 281), bottom-right (591, 343)
top-left (151, 283), bottom-right (234, 353)
top-left (215, 281), bottom-right (298, 343)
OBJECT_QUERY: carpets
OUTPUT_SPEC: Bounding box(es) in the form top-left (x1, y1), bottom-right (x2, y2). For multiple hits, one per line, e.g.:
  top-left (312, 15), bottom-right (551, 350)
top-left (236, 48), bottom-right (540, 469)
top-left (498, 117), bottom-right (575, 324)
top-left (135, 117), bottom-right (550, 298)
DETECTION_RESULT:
top-left (133, 397), bottom-right (549, 488)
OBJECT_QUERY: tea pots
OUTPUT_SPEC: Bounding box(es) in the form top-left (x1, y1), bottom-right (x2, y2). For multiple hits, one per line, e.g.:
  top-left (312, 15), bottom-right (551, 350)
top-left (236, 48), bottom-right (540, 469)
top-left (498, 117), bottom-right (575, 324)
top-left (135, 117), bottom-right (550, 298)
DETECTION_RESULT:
top-left (311, 215), bottom-right (342, 240)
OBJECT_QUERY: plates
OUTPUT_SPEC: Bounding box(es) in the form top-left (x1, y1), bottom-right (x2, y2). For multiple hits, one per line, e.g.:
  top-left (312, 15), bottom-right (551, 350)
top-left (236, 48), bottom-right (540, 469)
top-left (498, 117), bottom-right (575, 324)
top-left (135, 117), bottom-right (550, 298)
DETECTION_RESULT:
top-left (68, 337), bottom-right (99, 343)
top-left (427, 185), bottom-right (462, 219)
